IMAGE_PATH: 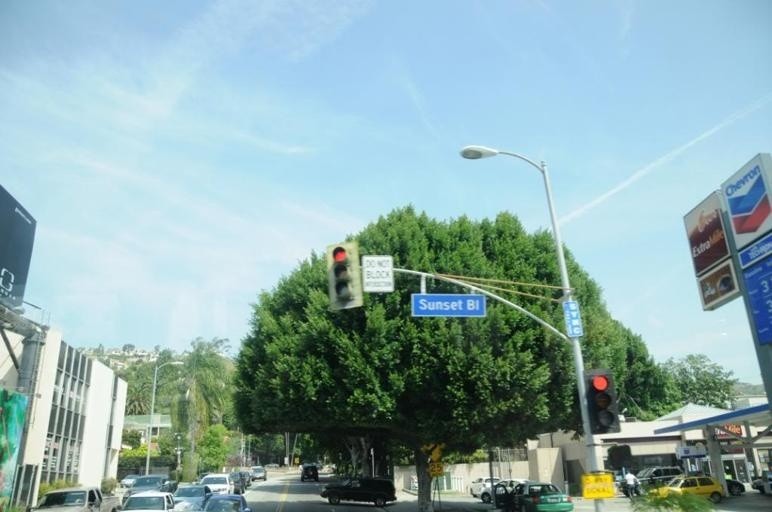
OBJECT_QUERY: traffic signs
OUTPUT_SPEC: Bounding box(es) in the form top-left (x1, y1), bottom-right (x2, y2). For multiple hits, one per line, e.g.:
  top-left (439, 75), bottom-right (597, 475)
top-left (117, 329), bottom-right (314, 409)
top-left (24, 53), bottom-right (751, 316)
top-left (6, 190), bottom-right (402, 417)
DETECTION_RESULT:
top-left (430, 463), bottom-right (443, 477)
top-left (411, 293), bottom-right (487, 317)
top-left (361, 255), bottom-right (395, 291)
top-left (563, 301), bottom-right (583, 338)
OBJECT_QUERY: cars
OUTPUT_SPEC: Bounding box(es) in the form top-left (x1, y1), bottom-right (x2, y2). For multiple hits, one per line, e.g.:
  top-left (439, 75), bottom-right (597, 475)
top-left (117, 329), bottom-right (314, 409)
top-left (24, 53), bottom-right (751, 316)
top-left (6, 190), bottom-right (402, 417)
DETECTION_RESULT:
top-left (650, 467), bottom-right (772, 502)
top-left (28, 465), bottom-right (270, 512)
top-left (469, 474), bottom-right (575, 512)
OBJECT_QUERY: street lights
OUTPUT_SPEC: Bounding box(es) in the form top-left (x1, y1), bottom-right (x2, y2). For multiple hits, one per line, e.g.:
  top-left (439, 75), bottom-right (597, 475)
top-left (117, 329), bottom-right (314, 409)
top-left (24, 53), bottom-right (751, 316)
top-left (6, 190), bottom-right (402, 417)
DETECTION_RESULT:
top-left (173, 432), bottom-right (184, 472)
top-left (458, 144), bottom-right (617, 512)
top-left (144, 361), bottom-right (188, 474)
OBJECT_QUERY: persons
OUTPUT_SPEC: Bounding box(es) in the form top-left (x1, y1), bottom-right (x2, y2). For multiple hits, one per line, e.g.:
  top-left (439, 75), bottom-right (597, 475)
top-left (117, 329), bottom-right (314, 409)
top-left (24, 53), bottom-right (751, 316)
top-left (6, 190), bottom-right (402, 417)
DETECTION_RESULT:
top-left (622, 469), bottom-right (639, 499)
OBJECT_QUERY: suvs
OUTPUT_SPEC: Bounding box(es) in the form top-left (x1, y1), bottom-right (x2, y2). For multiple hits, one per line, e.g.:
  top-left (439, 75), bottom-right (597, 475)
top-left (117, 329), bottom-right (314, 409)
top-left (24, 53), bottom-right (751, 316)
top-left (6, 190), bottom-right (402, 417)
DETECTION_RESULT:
top-left (617, 464), bottom-right (681, 498)
top-left (299, 462), bottom-right (320, 482)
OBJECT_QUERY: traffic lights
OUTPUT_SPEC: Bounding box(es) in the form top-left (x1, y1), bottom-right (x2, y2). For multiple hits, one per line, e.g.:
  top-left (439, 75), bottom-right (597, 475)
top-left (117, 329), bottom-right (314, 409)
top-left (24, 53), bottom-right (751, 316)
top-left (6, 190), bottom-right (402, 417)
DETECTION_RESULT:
top-left (583, 366), bottom-right (622, 436)
top-left (324, 240), bottom-right (363, 312)
top-left (609, 445), bottom-right (632, 468)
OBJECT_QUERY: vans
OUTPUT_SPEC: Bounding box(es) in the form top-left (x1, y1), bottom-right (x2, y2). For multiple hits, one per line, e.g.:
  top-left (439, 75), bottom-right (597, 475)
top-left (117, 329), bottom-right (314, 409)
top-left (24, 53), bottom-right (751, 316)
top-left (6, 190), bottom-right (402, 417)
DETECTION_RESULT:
top-left (319, 475), bottom-right (400, 509)
